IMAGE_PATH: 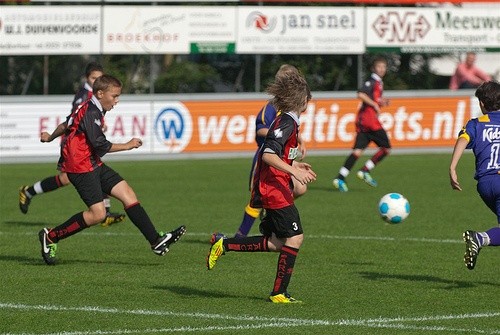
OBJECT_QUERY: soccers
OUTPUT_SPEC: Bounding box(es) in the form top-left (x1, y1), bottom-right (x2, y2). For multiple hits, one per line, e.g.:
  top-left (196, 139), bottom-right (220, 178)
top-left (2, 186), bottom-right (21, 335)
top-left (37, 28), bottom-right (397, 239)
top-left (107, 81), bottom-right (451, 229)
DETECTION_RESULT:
top-left (378, 193), bottom-right (410, 224)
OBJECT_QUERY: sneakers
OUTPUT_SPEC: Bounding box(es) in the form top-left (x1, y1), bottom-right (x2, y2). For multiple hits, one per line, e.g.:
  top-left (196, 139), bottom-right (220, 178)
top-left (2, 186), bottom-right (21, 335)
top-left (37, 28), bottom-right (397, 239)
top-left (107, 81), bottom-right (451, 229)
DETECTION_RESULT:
top-left (267, 290), bottom-right (302, 303)
top-left (99, 213), bottom-right (125, 228)
top-left (333, 178), bottom-right (348, 192)
top-left (152, 225), bottom-right (186, 256)
top-left (38, 228), bottom-right (58, 266)
top-left (206, 233), bottom-right (225, 270)
top-left (463, 229), bottom-right (482, 270)
top-left (19, 185), bottom-right (32, 214)
top-left (357, 171), bottom-right (376, 186)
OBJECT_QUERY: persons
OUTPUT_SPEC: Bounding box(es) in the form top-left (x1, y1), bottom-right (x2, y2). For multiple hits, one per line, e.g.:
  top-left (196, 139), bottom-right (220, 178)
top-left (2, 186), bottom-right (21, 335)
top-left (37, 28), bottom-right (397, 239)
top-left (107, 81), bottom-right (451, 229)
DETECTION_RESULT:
top-left (449, 81), bottom-right (500, 270)
top-left (449, 52), bottom-right (492, 88)
top-left (332, 55), bottom-right (392, 192)
top-left (206, 71), bottom-right (317, 305)
top-left (235, 65), bottom-right (307, 237)
top-left (19, 63), bottom-right (125, 226)
top-left (39, 75), bottom-right (186, 265)
